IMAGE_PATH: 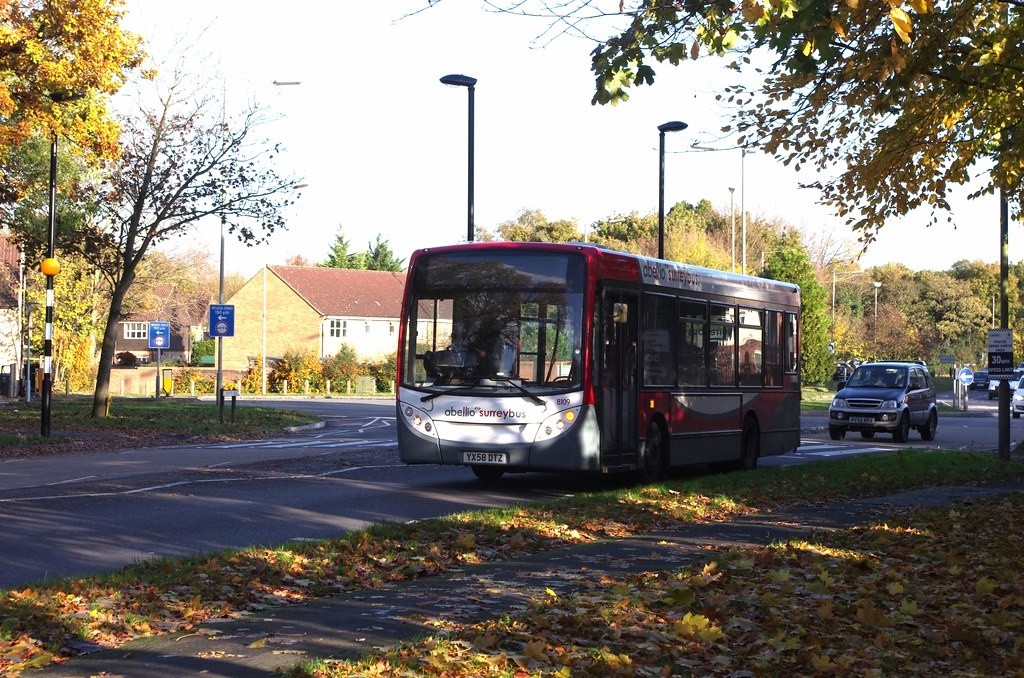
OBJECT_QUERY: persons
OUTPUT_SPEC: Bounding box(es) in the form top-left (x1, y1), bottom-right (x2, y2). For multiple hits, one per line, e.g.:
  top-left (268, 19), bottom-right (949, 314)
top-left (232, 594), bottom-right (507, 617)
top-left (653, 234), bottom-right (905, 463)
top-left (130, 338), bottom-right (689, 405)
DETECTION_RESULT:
top-left (451, 318), bottom-right (504, 370)
top-left (837, 362), bottom-right (851, 392)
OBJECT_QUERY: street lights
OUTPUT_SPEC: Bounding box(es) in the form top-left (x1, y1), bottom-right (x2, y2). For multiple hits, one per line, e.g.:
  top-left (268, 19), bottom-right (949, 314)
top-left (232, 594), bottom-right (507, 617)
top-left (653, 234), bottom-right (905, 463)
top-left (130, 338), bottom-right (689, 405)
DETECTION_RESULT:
top-left (24, 301), bottom-right (40, 403)
top-left (831, 270), bottom-right (864, 342)
top-left (740, 148), bottom-right (757, 276)
top-left (872, 281), bottom-right (882, 362)
top-left (439, 74), bottom-right (479, 240)
top-left (41, 258), bottom-right (61, 437)
top-left (657, 121), bottom-right (689, 260)
top-left (728, 186), bottom-right (735, 272)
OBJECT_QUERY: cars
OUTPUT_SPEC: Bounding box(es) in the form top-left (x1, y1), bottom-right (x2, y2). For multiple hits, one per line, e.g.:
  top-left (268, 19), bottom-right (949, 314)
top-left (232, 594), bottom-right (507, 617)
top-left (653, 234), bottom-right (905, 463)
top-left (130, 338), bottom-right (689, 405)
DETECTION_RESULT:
top-left (970, 371), bottom-right (989, 390)
top-left (987, 362), bottom-right (1024, 400)
top-left (1011, 375), bottom-right (1024, 419)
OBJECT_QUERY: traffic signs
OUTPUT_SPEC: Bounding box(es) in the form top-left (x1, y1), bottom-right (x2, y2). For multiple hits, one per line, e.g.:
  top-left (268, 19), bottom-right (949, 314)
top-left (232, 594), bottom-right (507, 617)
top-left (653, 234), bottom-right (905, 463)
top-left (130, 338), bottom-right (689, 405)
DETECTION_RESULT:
top-left (148, 322), bottom-right (170, 349)
top-left (208, 303), bottom-right (235, 337)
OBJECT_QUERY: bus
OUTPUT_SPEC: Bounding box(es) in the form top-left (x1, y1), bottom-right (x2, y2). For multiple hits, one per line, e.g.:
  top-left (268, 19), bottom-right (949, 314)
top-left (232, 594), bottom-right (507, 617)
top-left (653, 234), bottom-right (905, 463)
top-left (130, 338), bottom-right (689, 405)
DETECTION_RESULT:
top-left (393, 241), bottom-right (803, 493)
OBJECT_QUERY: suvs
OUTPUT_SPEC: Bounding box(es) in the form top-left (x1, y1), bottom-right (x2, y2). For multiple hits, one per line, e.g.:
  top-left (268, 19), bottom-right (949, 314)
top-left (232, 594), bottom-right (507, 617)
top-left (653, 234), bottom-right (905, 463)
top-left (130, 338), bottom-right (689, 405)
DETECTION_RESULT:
top-left (828, 361), bottom-right (940, 444)
